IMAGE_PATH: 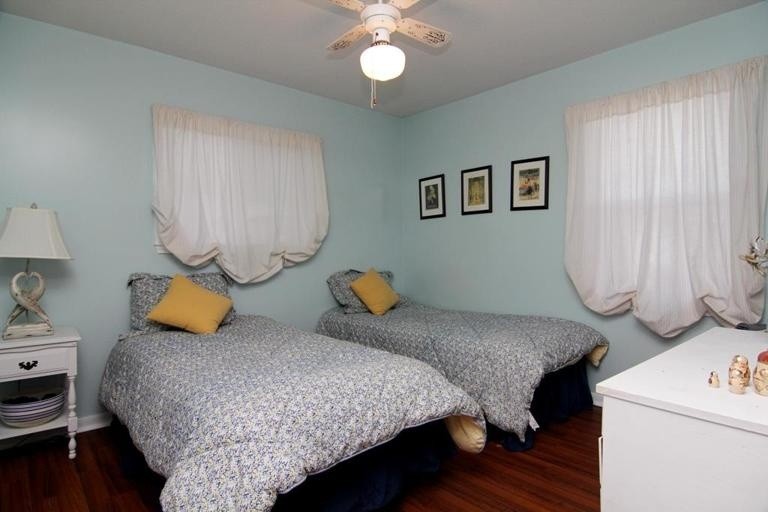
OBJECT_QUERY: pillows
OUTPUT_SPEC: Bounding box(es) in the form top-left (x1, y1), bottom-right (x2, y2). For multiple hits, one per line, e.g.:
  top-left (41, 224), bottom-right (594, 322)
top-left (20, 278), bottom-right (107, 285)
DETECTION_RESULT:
top-left (122, 270), bottom-right (238, 337)
top-left (322, 268), bottom-right (397, 315)
top-left (141, 272), bottom-right (235, 337)
top-left (347, 268), bottom-right (401, 317)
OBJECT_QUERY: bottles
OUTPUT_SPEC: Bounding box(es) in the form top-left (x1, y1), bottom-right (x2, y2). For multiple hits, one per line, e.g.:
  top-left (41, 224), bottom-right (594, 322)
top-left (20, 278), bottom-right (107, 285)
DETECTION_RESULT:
top-left (753, 351), bottom-right (768, 397)
top-left (729, 355), bottom-right (750, 387)
top-left (728, 369), bottom-right (745, 394)
top-left (708, 371), bottom-right (719, 388)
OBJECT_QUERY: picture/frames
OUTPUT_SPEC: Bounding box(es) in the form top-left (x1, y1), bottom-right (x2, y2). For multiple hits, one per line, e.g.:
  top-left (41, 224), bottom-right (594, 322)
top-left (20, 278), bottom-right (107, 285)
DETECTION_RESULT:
top-left (457, 164), bottom-right (493, 215)
top-left (416, 173), bottom-right (447, 221)
top-left (507, 156), bottom-right (551, 211)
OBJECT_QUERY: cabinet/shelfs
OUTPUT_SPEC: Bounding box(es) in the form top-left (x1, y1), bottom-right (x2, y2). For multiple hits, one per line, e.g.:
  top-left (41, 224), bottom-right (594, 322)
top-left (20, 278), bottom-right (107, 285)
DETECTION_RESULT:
top-left (592, 325), bottom-right (766, 512)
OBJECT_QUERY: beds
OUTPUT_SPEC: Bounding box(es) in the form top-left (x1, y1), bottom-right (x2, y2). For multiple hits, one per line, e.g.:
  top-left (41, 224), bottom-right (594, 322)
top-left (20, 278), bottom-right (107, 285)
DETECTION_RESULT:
top-left (312, 286), bottom-right (612, 449)
top-left (93, 267), bottom-right (491, 511)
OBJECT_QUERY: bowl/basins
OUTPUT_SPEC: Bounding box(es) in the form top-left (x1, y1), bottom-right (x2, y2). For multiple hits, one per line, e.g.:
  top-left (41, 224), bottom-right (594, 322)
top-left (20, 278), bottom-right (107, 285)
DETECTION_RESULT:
top-left (0, 386), bottom-right (66, 429)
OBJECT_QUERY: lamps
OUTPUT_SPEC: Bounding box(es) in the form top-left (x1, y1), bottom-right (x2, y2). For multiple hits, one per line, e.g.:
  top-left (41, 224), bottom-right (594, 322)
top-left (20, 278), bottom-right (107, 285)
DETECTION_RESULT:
top-left (358, 28), bottom-right (407, 109)
top-left (0, 200), bottom-right (74, 340)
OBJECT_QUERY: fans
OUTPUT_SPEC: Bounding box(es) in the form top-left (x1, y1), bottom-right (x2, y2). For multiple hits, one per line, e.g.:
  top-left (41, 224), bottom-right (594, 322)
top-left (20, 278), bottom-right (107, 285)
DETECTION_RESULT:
top-left (322, 0), bottom-right (456, 55)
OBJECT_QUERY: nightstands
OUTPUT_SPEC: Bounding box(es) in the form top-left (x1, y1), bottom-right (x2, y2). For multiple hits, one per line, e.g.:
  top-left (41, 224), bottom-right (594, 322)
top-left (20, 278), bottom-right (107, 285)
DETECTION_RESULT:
top-left (0, 321), bottom-right (83, 460)
